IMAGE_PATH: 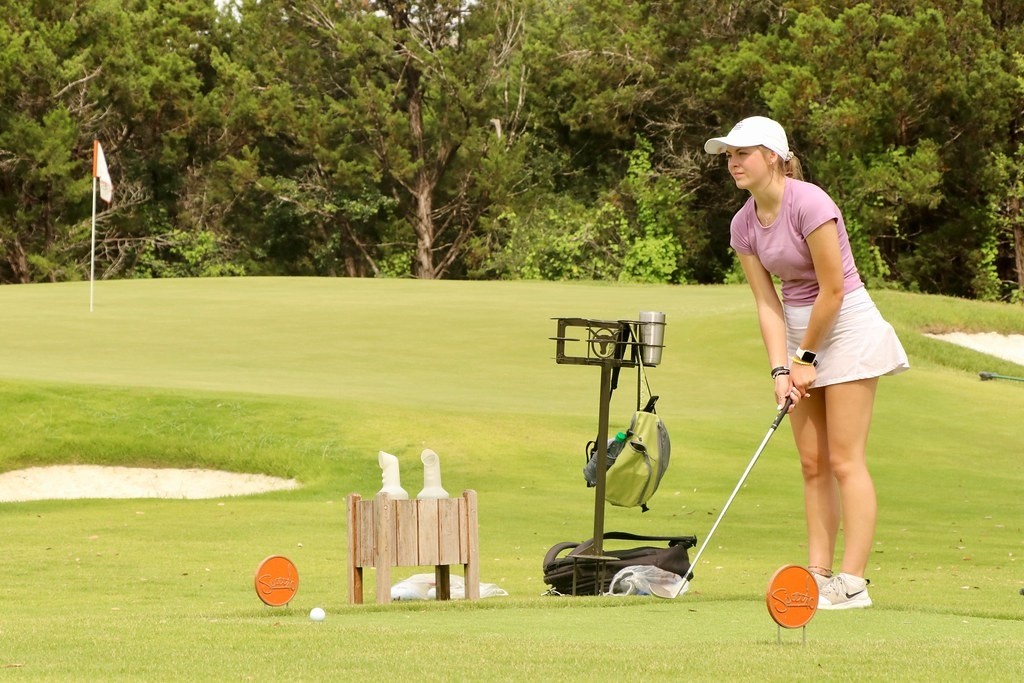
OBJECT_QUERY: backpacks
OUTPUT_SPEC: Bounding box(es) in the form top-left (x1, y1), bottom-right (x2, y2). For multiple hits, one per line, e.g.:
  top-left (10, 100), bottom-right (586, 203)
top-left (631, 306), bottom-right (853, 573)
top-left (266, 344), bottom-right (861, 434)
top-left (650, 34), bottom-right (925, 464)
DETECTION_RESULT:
top-left (587, 325), bottom-right (670, 506)
top-left (543, 536), bottom-right (696, 595)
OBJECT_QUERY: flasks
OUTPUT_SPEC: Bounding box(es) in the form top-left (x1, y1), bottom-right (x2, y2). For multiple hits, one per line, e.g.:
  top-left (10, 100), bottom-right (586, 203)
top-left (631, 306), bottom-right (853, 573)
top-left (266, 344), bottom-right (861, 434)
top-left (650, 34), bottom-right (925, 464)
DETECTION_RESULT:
top-left (639, 311), bottom-right (665, 365)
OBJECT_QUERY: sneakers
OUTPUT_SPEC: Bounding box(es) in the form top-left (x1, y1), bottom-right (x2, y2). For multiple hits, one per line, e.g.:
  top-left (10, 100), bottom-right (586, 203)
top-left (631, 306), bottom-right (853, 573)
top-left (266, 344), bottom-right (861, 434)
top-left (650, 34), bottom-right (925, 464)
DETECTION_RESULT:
top-left (809, 572), bottom-right (872, 610)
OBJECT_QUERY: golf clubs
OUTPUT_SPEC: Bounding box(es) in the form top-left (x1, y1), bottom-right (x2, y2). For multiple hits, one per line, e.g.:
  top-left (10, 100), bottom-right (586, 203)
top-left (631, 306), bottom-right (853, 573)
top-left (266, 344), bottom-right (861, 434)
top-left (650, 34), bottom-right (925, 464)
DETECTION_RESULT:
top-left (645, 396), bottom-right (792, 598)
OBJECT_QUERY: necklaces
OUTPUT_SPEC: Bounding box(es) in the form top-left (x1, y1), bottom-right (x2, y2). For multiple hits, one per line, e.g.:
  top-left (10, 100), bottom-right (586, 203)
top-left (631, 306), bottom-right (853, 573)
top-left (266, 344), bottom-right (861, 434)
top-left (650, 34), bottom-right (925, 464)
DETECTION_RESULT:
top-left (756, 204), bottom-right (776, 228)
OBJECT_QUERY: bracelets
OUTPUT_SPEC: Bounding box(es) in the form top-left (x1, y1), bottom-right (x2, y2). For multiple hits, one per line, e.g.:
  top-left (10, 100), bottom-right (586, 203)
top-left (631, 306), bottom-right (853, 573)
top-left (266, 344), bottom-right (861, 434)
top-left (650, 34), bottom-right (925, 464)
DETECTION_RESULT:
top-left (770, 366), bottom-right (790, 379)
top-left (792, 357), bottom-right (812, 366)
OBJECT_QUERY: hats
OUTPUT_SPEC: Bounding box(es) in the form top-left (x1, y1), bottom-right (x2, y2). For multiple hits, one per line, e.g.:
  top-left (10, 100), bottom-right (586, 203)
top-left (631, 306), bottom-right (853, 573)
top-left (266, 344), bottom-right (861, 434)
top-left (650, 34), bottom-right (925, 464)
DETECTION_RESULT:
top-left (704, 116), bottom-right (789, 163)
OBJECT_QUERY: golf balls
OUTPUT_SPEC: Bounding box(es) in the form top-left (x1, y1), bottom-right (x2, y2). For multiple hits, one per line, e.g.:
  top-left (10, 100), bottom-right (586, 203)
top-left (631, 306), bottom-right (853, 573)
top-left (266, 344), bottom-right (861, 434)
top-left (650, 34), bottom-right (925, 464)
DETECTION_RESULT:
top-left (310, 607), bottom-right (325, 621)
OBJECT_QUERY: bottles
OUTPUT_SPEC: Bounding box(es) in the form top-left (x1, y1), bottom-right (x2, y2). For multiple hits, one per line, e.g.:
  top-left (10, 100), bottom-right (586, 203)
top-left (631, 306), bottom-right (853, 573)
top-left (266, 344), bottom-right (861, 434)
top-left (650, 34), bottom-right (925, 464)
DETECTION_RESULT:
top-left (582, 433), bottom-right (625, 483)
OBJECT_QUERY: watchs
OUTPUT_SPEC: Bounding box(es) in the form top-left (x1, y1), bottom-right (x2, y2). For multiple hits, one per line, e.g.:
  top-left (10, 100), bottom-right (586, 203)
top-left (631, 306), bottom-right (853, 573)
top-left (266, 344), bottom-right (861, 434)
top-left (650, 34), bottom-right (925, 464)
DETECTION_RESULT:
top-left (794, 347), bottom-right (822, 367)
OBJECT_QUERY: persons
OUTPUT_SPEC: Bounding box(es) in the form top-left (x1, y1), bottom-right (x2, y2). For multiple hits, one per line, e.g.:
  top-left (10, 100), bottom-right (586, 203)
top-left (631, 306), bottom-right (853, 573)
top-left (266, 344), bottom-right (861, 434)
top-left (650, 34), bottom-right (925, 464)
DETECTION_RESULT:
top-left (705, 116), bottom-right (911, 610)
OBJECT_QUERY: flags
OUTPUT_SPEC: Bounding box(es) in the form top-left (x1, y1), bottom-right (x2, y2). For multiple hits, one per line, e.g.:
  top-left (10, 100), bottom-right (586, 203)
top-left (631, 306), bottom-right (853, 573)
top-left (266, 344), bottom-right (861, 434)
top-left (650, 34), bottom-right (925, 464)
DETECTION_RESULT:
top-left (92, 139), bottom-right (113, 202)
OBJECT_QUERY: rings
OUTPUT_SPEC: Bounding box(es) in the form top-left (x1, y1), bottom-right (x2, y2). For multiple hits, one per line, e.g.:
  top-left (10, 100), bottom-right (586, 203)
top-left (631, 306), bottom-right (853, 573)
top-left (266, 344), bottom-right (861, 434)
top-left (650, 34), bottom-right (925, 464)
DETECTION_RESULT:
top-left (777, 396), bottom-right (785, 399)
